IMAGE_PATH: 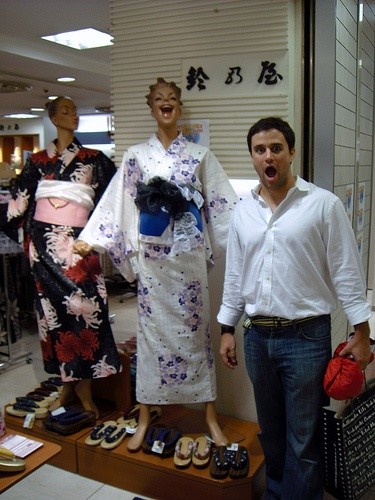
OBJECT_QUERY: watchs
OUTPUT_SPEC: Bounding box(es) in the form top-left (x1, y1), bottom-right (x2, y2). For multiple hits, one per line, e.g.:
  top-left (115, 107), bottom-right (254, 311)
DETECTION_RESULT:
top-left (219, 325), bottom-right (235, 336)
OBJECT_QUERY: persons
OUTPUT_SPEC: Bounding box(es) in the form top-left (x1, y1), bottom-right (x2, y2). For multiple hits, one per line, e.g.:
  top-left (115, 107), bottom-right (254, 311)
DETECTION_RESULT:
top-left (5, 77), bottom-right (240, 449)
top-left (210, 117), bottom-right (369, 500)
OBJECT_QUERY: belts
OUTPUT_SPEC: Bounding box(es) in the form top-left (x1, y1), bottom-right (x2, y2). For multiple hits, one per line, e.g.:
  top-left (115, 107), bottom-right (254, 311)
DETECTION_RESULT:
top-left (242, 314), bottom-right (319, 329)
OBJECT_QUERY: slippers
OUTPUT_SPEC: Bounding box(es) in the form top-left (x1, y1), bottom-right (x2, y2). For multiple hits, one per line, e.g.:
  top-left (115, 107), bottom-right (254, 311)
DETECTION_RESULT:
top-left (5, 376), bottom-right (64, 419)
top-left (142, 424), bottom-right (168, 451)
top-left (100, 424), bottom-right (127, 449)
top-left (173, 437), bottom-right (193, 469)
top-left (210, 446), bottom-right (228, 479)
top-left (84, 420), bottom-right (117, 446)
top-left (192, 436), bottom-right (210, 469)
top-left (116, 403), bottom-right (141, 424)
top-left (229, 446), bottom-right (250, 479)
top-left (125, 406), bottom-right (161, 434)
top-left (43, 406), bottom-right (81, 431)
top-left (159, 428), bottom-right (179, 458)
top-left (55, 411), bottom-right (96, 434)
top-left (0, 448), bottom-right (25, 471)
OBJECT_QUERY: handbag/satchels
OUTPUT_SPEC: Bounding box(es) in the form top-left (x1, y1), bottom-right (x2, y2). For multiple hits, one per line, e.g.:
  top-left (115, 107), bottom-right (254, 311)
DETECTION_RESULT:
top-left (322, 368), bottom-right (375, 500)
